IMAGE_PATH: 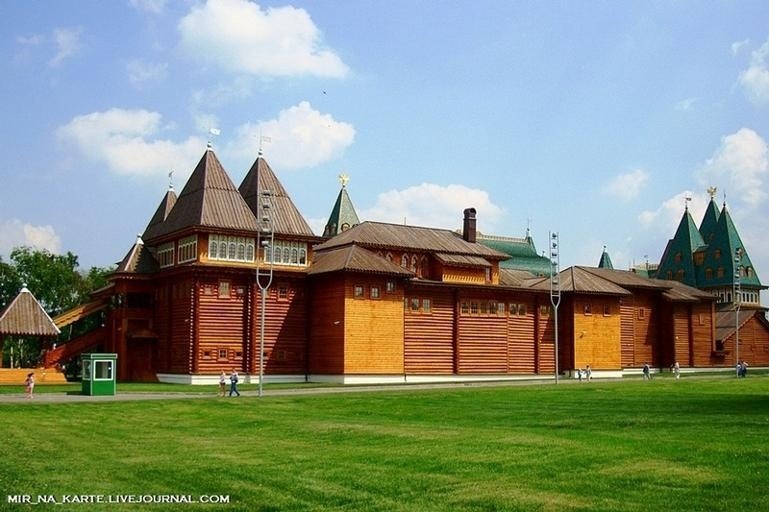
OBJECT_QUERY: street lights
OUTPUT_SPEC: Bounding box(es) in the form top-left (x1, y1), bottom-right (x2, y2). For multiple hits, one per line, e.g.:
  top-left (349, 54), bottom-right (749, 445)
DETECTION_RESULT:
top-left (733, 250), bottom-right (740, 378)
top-left (256, 185), bottom-right (278, 397)
top-left (548, 229), bottom-right (561, 385)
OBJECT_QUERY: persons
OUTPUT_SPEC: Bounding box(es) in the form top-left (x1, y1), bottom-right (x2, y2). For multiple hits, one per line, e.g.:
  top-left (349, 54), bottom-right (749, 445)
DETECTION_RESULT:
top-left (642, 361), bottom-right (650, 381)
top-left (578, 368), bottom-right (584, 381)
top-left (218, 369), bottom-right (226, 397)
top-left (583, 364), bottom-right (592, 382)
top-left (736, 362), bottom-right (748, 378)
top-left (673, 362), bottom-right (681, 379)
top-left (26, 372), bottom-right (34, 399)
top-left (227, 368), bottom-right (240, 397)
